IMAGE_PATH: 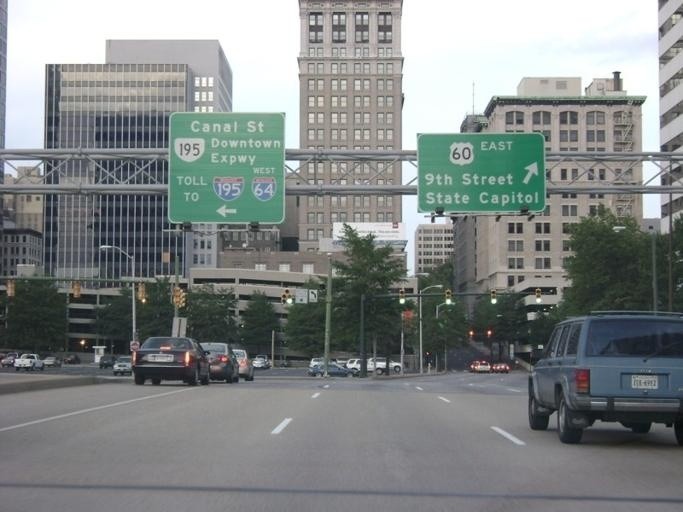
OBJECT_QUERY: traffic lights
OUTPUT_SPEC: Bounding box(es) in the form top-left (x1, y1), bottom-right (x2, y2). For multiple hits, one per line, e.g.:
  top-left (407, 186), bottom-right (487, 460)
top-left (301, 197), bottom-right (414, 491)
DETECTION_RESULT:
top-left (445, 290), bottom-right (451, 304)
top-left (174, 287), bottom-right (189, 309)
top-left (283, 289), bottom-right (293, 304)
top-left (490, 289), bottom-right (497, 304)
top-left (535, 288), bottom-right (543, 304)
top-left (399, 290), bottom-right (404, 304)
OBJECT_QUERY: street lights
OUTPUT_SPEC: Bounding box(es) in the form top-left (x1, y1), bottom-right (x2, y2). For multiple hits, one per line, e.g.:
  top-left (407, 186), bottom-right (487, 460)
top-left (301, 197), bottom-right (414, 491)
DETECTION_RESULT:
top-left (99, 245), bottom-right (137, 342)
top-left (400, 273), bottom-right (429, 374)
top-left (435, 302), bottom-right (455, 376)
top-left (418, 284), bottom-right (443, 376)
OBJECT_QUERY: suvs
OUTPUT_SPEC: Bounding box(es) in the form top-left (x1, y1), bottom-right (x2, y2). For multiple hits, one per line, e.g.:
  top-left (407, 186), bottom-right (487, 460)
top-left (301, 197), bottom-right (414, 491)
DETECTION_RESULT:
top-left (528, 309), bottom-right (682, 444)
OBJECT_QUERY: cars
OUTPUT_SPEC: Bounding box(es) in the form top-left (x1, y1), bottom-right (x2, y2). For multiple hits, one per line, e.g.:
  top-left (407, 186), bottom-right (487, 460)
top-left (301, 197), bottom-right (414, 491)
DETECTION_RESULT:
top-left (0, 352), bottom-right (80, 371)
top-left (100, 354), bottom-right (132, 376)
top-left (309, 357), bottom-right (405, 378)
top-left (468, 361), bottom-right (510, 374)
top-left (134, 336), bottom-right (271, 386)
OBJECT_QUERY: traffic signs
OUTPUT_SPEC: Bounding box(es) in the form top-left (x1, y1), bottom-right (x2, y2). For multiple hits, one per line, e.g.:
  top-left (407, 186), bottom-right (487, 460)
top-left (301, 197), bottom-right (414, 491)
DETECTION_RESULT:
top-left (417, 133), bottom-right (546, 215)
top-left (167, 111), bottom-right (287, 224)
